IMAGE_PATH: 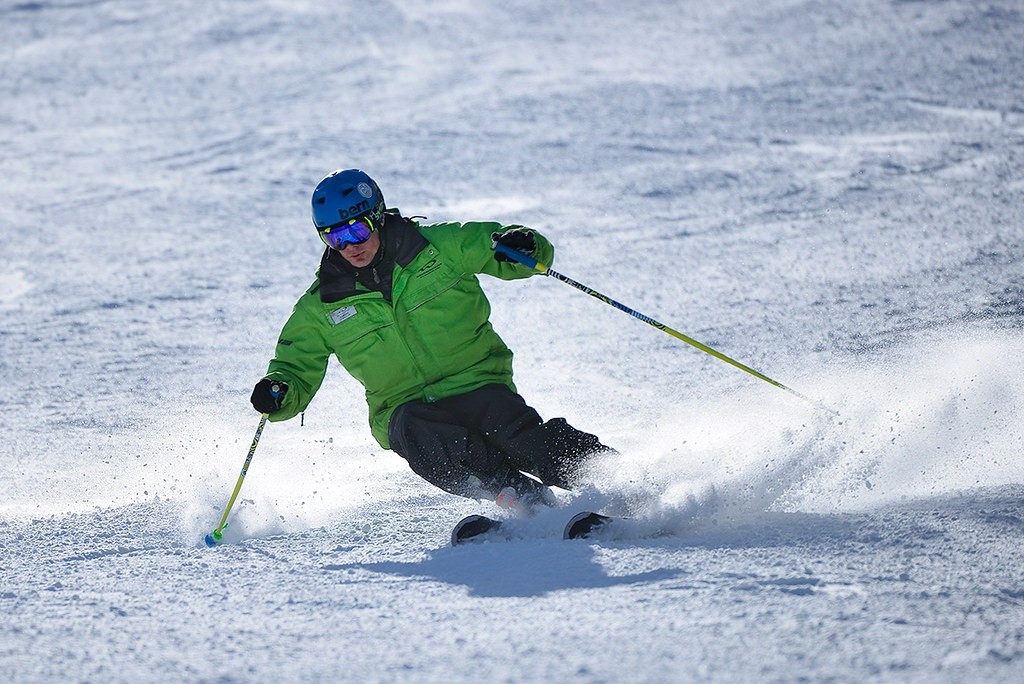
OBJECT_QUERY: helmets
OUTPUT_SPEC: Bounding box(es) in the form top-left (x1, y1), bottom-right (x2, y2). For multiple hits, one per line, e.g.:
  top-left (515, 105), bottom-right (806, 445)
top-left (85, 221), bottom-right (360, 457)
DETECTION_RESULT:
top-left (311, 168), bottom-right (383, 231)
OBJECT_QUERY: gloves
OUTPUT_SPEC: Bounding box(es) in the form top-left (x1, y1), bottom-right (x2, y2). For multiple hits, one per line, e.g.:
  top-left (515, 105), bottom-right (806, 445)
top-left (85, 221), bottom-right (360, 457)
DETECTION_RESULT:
top-left (250, 378), bottom-right (289, 414)
top-left (491, 231), bottom-right (535, 263)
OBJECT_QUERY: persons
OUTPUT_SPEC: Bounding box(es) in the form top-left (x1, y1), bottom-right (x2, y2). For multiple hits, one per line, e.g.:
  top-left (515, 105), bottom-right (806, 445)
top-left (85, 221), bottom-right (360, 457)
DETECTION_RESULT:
top-left (249, 170), bottom-right (626, 517)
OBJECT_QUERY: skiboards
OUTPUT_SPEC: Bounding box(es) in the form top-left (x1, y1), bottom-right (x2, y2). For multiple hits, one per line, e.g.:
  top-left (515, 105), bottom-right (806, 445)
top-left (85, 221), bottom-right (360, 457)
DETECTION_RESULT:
top-left (450, 512), bottom-right (633, 551)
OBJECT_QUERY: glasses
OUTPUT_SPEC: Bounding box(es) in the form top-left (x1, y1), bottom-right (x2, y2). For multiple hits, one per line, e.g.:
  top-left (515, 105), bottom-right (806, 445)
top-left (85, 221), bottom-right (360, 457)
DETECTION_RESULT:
top-left (319, 217), bottom-right (375, 250)
